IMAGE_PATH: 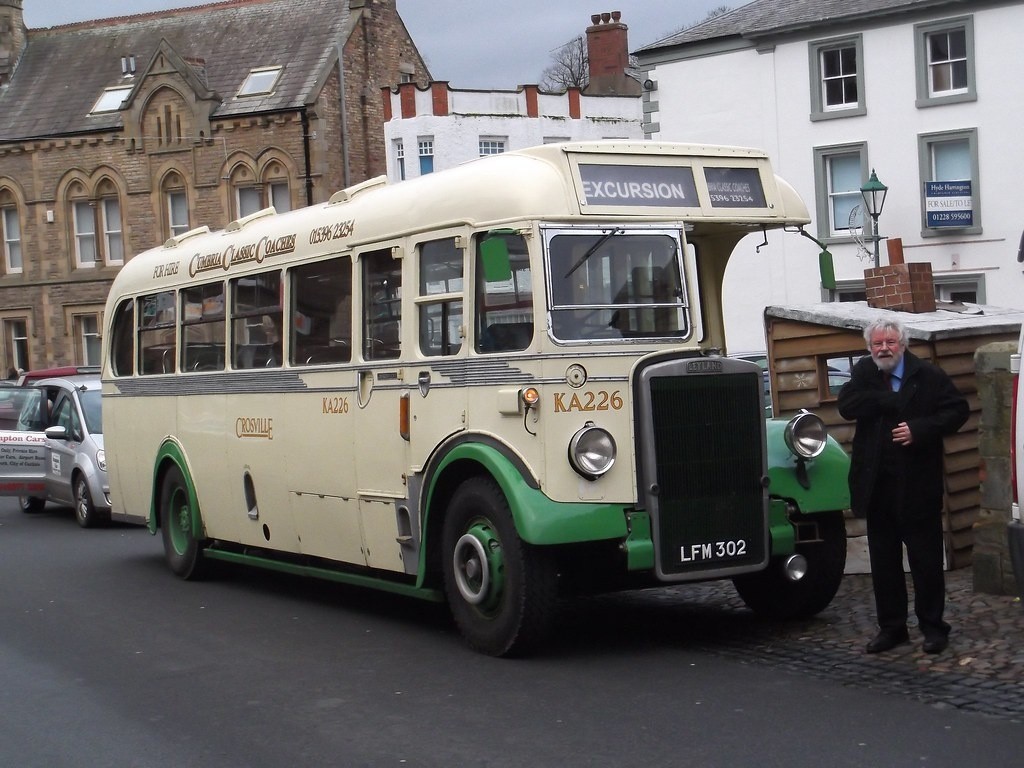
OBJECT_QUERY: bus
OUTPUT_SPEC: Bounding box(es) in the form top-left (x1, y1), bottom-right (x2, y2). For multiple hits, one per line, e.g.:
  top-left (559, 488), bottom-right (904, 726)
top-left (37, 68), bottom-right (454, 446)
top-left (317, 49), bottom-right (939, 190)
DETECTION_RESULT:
top-left (101, 139), bottom-right (851, 658)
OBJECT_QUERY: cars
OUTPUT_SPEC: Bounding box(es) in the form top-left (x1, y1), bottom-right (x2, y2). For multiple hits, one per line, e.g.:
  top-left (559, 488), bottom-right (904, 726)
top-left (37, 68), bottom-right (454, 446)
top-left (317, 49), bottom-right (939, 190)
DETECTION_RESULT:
top-left (0, 366), bottom-right (112, 528)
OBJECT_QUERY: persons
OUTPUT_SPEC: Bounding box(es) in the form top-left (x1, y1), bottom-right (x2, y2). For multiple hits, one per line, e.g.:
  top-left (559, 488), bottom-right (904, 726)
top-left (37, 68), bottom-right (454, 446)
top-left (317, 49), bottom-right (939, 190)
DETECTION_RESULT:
top-left (838, 316), bottom-right (971, 652)
top-left (7, 367), bottom-right (20, 380)
top-left (35, 399), bottom-right (71, 434)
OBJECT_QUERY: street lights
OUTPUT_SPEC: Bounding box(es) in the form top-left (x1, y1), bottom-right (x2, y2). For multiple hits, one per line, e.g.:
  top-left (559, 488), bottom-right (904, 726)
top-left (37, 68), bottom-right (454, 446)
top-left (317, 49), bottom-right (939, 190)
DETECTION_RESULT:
top-left (860, 168), bottom-right (888, 268)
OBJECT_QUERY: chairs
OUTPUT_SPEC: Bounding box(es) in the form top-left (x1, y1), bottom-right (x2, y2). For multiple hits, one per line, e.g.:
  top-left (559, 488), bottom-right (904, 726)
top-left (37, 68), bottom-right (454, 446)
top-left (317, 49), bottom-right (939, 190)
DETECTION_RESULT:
top-left (237, 345), bottom-right (272, 369)
top-left (368, 341), bottom-right (401, 359)
top-left (194, 351), bottom-right (225, 371)
top-left (311, 345), bottom-right (351, 363)
top-left (481, 322), bottom-right (534, 351)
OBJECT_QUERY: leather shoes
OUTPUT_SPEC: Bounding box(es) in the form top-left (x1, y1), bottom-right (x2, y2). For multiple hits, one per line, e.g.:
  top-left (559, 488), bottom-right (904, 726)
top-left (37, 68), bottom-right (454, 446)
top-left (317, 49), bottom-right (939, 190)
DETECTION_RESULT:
top-left (922, 635), bottom-right (948, 655)
top-left (866, 627), bottom-right (910, 653)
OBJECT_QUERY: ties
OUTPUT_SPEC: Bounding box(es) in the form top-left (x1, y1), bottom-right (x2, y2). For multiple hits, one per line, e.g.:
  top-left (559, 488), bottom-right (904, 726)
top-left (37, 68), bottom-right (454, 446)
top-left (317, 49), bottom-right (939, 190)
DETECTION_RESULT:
top-left (885, 373), bottom-right (893, 394)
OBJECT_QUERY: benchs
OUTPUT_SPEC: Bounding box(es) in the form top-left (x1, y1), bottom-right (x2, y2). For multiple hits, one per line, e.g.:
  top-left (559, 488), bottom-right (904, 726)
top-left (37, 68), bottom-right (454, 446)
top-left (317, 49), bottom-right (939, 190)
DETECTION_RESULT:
top-left (161, 347), bottom-right (225, 373)
